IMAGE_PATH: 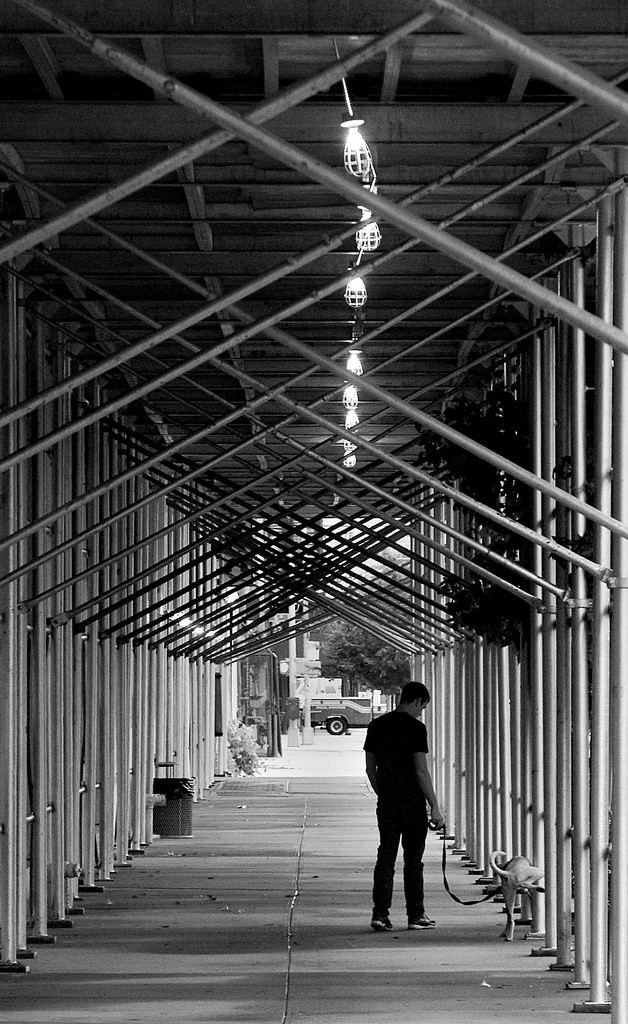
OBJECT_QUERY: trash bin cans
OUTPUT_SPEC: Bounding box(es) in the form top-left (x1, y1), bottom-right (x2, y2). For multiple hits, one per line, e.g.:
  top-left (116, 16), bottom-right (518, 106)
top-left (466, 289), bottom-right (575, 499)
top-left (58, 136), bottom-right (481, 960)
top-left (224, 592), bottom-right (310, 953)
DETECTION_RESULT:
top-left (152, 776), bottom-right (197, 838)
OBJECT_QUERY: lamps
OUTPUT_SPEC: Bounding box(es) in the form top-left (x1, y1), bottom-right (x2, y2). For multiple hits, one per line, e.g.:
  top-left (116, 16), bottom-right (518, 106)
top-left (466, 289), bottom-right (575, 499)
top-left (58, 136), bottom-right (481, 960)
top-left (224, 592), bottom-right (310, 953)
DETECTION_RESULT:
top-left (341, 111), bottom-right (373, 176)
top-left (342, 380), bottom-right (359, 468)
top-left (355, 182), bottom-right (383, 252)
top-left (343, 260), bottom-right (368, 308)
top-left (347, 349), bottom-right (364, 377)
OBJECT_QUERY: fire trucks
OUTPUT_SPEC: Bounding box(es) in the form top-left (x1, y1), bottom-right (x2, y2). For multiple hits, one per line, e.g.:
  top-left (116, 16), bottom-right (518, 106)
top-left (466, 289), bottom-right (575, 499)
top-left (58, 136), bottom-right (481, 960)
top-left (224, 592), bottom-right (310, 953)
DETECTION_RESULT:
top-left (295, 678), bottom-right (396, 736)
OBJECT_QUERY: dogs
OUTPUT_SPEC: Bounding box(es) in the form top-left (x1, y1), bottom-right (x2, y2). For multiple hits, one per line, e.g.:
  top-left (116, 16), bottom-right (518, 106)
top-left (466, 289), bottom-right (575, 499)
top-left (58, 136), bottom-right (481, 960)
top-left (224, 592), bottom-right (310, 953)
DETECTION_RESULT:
top-left (489, 850), bottom-right (546, 942)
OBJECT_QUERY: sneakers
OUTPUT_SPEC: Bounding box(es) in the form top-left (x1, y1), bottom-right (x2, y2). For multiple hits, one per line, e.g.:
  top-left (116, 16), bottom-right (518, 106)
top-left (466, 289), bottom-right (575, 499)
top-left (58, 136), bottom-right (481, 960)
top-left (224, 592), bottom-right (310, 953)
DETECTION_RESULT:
top-left (408, 913), bottom-right (437, 929)
top-left (370, 911), bottom-right (393, 930)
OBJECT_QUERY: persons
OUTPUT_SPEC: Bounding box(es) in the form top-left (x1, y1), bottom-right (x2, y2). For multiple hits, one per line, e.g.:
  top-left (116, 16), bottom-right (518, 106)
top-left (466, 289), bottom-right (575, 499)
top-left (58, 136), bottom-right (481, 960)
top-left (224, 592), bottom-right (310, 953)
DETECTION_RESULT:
top-left (362, 681), bottom-right (444, 931)
